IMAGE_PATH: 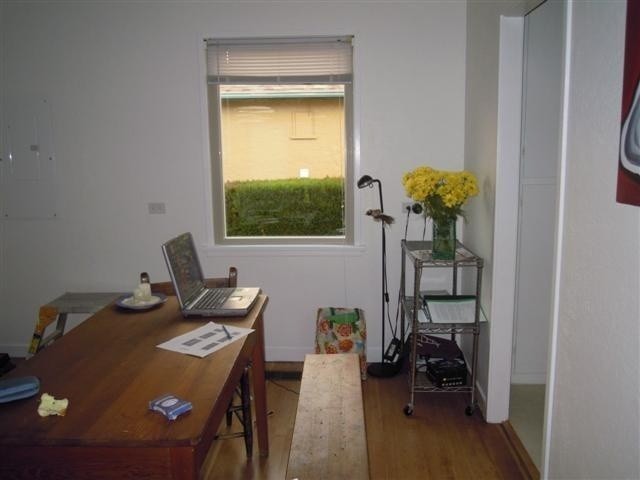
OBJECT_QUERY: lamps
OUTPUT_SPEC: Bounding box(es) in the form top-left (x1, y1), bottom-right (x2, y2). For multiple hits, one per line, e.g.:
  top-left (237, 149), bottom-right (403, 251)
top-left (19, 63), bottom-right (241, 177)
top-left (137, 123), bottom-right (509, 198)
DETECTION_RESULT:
top-left (357, 174), bottom-right (399, 379)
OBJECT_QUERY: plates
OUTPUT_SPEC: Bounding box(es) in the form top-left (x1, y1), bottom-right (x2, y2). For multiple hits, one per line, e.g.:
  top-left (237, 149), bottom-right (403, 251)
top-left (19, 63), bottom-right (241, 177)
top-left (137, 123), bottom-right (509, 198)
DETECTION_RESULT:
top-left (114, 291), bottom-right (168, 312)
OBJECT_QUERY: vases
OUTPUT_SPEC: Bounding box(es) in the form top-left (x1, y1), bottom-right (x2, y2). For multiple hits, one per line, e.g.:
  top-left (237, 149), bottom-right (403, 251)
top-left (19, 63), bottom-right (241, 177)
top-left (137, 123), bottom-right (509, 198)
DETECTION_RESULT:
top-left (432, 215), bottom-right (456, 260)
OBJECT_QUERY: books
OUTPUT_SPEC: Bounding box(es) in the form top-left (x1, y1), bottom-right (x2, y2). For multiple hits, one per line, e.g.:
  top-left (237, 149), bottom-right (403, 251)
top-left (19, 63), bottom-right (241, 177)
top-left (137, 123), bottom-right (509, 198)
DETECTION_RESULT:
top-left (418, 287), bottom-right (488, 326)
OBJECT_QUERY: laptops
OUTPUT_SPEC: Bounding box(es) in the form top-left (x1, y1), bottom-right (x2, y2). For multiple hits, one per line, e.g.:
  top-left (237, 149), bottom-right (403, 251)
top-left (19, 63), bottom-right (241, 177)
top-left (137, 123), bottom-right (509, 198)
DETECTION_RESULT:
top-left (162, 232), bottom-right (262, 317)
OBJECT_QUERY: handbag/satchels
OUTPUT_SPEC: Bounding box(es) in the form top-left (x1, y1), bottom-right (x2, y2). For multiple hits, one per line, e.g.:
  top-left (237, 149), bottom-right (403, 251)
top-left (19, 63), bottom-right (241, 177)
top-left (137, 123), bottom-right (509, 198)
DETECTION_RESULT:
top-left (316, 306), bottom-right (368, 382)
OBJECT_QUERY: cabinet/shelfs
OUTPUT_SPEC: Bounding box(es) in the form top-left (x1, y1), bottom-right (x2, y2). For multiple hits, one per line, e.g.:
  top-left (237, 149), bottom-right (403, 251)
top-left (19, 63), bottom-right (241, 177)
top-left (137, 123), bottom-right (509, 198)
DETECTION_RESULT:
top-left (401, 238), bottom-right (484, 416)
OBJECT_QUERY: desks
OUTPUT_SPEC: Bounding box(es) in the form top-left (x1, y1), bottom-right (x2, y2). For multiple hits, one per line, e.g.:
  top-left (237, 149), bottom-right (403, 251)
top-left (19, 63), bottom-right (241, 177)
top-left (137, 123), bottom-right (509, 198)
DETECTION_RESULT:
top-left (0, 292), bottom-right (269, 480)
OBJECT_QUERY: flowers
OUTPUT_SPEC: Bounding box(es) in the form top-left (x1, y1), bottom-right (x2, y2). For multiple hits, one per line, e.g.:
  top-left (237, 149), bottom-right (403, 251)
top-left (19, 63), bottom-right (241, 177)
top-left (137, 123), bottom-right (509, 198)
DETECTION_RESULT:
top-left (401, 166), bottom-right (479, 253)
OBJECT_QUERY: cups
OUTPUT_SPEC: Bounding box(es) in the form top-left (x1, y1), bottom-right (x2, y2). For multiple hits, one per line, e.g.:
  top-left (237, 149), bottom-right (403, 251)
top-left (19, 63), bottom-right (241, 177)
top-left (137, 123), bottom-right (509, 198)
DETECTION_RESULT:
top-left (132, 282), bottom-right (153, 302)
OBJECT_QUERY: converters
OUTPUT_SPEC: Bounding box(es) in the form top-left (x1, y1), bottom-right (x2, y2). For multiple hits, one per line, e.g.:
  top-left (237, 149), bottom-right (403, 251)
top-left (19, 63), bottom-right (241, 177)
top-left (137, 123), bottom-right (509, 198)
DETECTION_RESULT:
top-left (384, 337), bottom-right (400, 362)
top-left (412, 203), bottom-right (422, 214)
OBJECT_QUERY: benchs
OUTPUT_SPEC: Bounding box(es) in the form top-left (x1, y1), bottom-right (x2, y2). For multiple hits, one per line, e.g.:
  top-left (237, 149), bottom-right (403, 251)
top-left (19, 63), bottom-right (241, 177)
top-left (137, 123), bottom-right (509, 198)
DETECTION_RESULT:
top-left (285, 353), bottom-right (370, 480)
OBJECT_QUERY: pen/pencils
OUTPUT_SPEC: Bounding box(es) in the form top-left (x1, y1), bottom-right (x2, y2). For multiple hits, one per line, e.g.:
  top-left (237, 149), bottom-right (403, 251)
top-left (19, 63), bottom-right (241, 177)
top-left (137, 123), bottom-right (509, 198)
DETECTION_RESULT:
top-left (222, 325), bottom-right (231, 339)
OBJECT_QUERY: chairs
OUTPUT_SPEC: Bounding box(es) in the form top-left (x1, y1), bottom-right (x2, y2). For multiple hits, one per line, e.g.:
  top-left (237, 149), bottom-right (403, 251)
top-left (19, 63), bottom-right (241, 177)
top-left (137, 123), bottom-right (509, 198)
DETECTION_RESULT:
top-left (140, 267), bottom-right (253, 461)
